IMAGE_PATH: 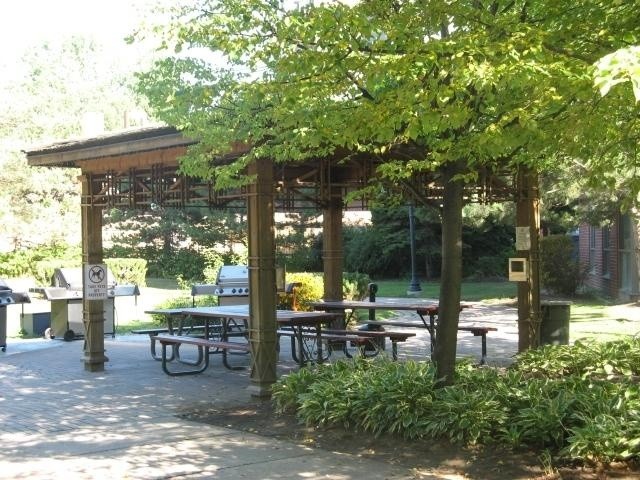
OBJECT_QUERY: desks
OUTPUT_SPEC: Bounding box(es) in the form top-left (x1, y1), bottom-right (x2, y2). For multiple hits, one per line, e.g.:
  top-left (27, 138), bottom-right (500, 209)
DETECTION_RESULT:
top-left (145, 304), bottom-right (346, 370)
top-left (315, 301), bottom-right (475, 359)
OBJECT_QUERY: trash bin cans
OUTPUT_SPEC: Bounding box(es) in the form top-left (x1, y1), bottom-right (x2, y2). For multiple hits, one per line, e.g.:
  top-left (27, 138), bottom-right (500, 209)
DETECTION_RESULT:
top-left (540, 300), bottom-right (574, 347)
